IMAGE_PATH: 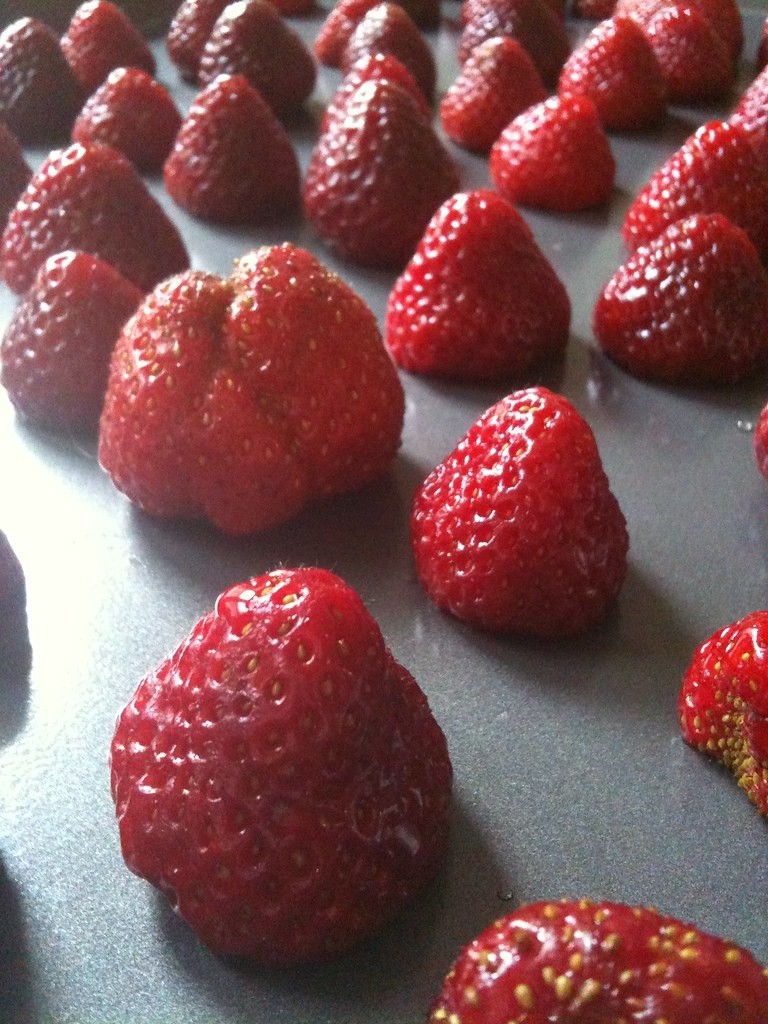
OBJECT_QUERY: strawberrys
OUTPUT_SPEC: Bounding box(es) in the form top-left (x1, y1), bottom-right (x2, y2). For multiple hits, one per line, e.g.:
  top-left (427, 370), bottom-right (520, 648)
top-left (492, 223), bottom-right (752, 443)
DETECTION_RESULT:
top-left (0, 0), bottom-right (768, 1024)
